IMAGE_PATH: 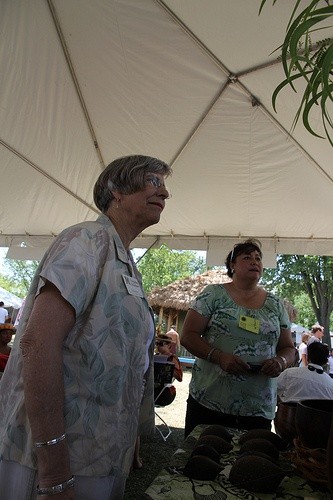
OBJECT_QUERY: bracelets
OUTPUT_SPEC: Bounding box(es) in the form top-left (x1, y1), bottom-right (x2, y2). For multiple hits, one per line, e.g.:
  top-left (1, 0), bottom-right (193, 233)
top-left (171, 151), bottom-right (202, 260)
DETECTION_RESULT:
top-left (279, 356), bottom-right (287, 369)
top-left (35, 432), bottom-right (67, 448)
top-left (207, 348), bottom-right (215, 362)
top-left (274, 358), bottom-right (282, 371)
top-left (36, 474), bottom-right (75, 497)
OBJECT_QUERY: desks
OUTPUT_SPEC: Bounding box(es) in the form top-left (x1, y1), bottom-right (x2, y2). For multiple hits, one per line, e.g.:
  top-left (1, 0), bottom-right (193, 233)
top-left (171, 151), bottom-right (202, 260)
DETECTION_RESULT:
top-left (144, 424), bottom-right (333, 500)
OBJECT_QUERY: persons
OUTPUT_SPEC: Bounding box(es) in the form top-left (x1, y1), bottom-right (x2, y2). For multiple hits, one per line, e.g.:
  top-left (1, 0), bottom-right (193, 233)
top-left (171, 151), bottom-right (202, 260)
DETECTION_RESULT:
top-left (180, 238), bottom-right (296, 438)
top-left (0, 301), bottom-right (8, 323)
top-left (298, 325), bottom-right (333, 378)
top-left (277, 342), bottom-right (333, 404)
top-left (154, 334), bottom-right (183, 407)
top-left (1, 154), bottom-right (172, 500)
top-left (0, 324), bottom-right (17, 373)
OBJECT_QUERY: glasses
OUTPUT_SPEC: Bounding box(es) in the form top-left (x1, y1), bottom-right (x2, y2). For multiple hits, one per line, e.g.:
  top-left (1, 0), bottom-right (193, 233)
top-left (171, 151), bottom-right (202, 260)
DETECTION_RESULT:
top-left (155, 341), bottom-right (167, 347)
top-left (308, 364), bottom-right (325, 374)
top-left (230, 243), bottom-right (244, 261)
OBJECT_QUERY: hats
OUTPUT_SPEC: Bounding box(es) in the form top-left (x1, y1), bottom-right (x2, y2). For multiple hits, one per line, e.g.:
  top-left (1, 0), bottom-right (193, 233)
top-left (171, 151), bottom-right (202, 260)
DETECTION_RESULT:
top-left (155, 334), bottom-right (176, 344)
